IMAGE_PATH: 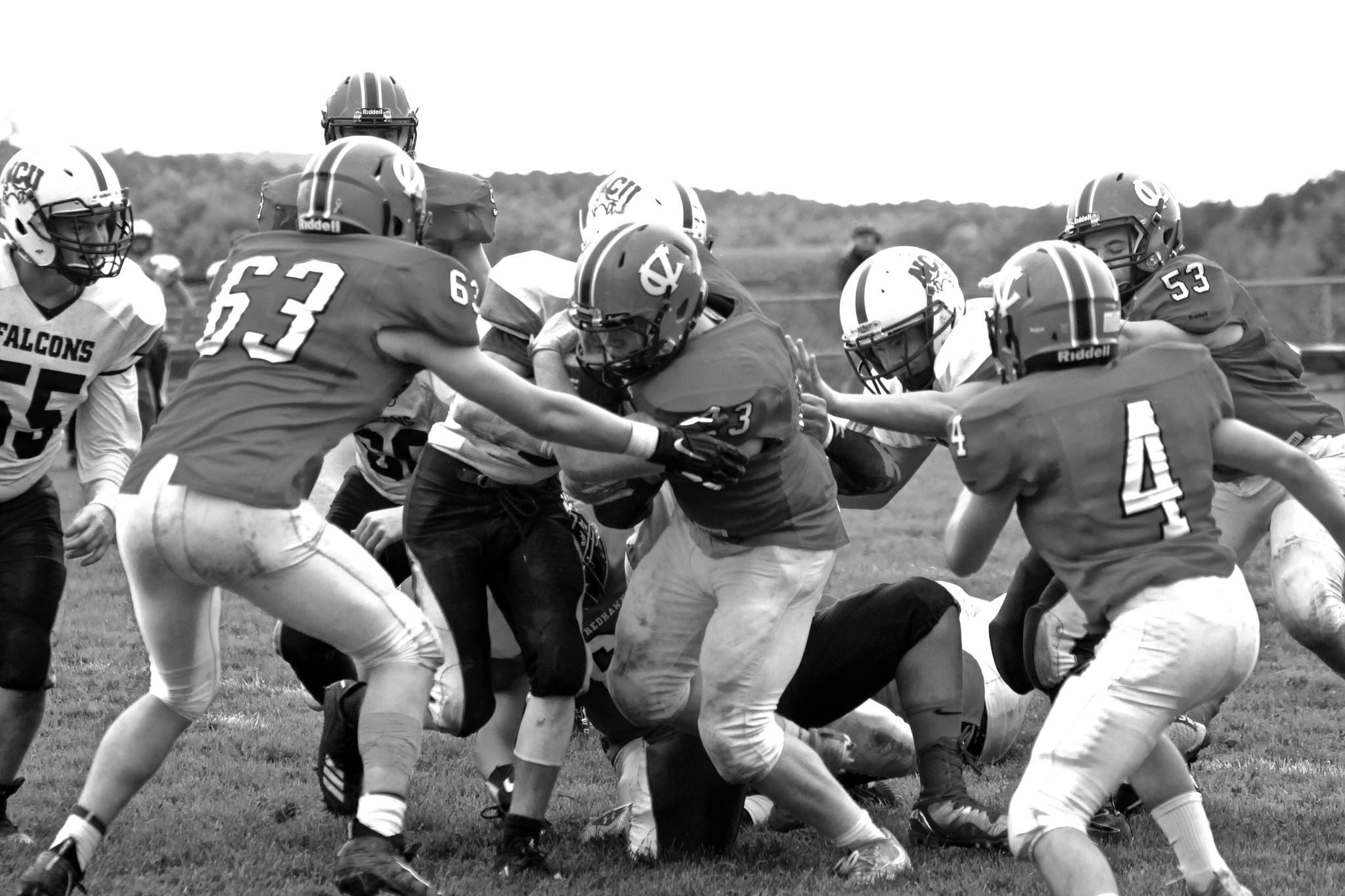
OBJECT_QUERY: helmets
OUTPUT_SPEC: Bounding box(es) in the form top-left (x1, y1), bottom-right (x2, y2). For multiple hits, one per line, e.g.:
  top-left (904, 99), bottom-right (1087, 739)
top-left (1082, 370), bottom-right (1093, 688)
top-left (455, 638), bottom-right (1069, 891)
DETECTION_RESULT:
top-left (567, 223), bottom-right (711, 395)
top-left (0, 141), bottom-right (134, 283)
top-left (298, 135), bottom-right (432, 247)
top-left (577, 170), bottom-right (714, 254)
top-left (1061, 172), bottom-right (1184, 302)
top-left (321, 72), bottom-right (419, 162)
top-left (124, 220), bottom-right (154, 253)
top-left (978, 239), bottom-right (1119, 384)
top-left (838, 245), bottom-right (965, 396)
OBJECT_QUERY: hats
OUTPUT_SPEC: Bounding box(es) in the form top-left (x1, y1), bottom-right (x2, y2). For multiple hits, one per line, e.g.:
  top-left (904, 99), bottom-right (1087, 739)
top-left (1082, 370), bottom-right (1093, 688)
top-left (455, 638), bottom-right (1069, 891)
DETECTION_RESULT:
top-left (852, 225), bottom-right (883, 246)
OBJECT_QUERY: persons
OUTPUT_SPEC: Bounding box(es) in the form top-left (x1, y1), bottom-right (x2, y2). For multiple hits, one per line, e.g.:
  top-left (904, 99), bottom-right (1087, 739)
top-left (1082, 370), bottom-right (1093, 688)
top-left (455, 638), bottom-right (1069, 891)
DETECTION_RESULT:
top-left (1, 73), bottom-right (1345, 895)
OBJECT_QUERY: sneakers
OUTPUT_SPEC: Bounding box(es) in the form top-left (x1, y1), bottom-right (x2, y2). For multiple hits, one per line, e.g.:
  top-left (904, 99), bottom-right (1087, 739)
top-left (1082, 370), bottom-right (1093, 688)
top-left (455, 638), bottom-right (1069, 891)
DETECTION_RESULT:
top-left (1087, 807), bottom-right (1134, 844)
top-left (577, 804), bottom-right (631, 843)
top-left (493, 813), bottom-right (559, 877)
top-left (1115, 713), bottom-right (1212, 814)
top-left (829, 826), bottom-right (913, 891)
top-left (907, 792), bottom-right (1011, 852)
top-left (330, 819), bottom-right (445, 896)
top-left (15, 835), bottom-right (89, 896)
top-left (0, 777), bottom-right (35, 847)
top-left (768, 726), bottom-right (859, 832)
top-left (316, 678), bottom-right (369, 819)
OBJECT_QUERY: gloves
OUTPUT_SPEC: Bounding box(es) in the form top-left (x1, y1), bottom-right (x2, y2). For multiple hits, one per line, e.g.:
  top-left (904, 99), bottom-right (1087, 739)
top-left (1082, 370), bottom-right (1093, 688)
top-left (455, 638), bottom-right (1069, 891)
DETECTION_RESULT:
top-left (623, 413), bottom-right (751, 493)
